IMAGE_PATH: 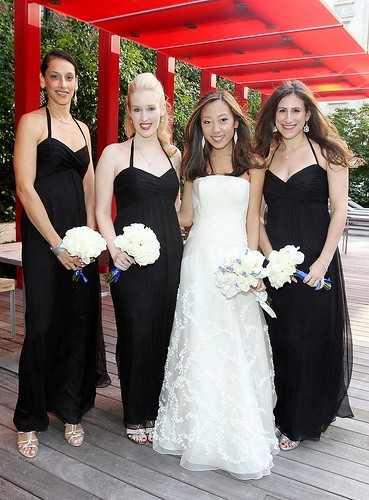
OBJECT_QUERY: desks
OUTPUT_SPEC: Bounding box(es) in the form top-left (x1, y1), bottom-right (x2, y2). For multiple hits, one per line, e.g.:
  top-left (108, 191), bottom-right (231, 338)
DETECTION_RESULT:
top-left (0, 242), bottom-right (26, 338)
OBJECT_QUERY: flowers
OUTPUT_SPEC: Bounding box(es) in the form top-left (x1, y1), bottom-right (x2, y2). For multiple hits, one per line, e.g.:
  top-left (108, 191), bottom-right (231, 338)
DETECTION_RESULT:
top-left (216, 249), bottom-right (272, 306)
top-left (104, 223), bottom-right (161, 283)
top-left (59, 226), bottom-right (107, 282)
top-left (266, 245), bottom-right (333, 291)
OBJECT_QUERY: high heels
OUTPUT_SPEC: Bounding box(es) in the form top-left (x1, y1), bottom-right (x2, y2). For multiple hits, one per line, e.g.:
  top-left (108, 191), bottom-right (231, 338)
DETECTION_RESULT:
top-left (279, 433), bottom-right (300, 450)
top-left (64, 423), bottom-right (84, 447)
top-left (146, 427), bottom-right (160, 443)
top-left (16, 430), bottom-right (38, 458)
top-left (126, 426), bottom-right (147, 445)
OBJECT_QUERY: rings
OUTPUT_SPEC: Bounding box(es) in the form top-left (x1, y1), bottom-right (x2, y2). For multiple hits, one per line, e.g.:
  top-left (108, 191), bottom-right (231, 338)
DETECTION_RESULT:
top-left (122, 258), bottom-right (127, 264)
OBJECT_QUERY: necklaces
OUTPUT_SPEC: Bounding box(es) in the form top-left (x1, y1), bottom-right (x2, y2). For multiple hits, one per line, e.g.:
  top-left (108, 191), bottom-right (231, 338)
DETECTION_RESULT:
top-left (48, 107), bottom-right (74, 125)
top-left (136, 145), bottom-right (164, 166)
top-left (280, 141), bottom-right (305, 160)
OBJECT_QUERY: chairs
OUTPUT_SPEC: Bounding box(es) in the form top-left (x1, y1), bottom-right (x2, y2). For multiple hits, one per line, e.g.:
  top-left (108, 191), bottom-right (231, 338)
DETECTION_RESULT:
top-left (0, 277), bottom-right (15, 338)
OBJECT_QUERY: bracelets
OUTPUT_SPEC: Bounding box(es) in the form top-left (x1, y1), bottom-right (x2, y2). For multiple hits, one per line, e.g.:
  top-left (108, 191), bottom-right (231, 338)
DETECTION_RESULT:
top-left (50, 240), bottom-right (66, 256)
top-left (317, 258), bottom-right (328, 273)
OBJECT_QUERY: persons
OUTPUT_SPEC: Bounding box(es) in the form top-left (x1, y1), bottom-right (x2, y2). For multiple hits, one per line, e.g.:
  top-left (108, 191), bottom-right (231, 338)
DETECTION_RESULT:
top-left (94, 72), bottom-right (184, 444)
top-left (153, 90), bottom-right (281, 479)
top-left (253, 80), bottom-right (355, 452)
top-left (13, 49), bottom-right (95, 457)
